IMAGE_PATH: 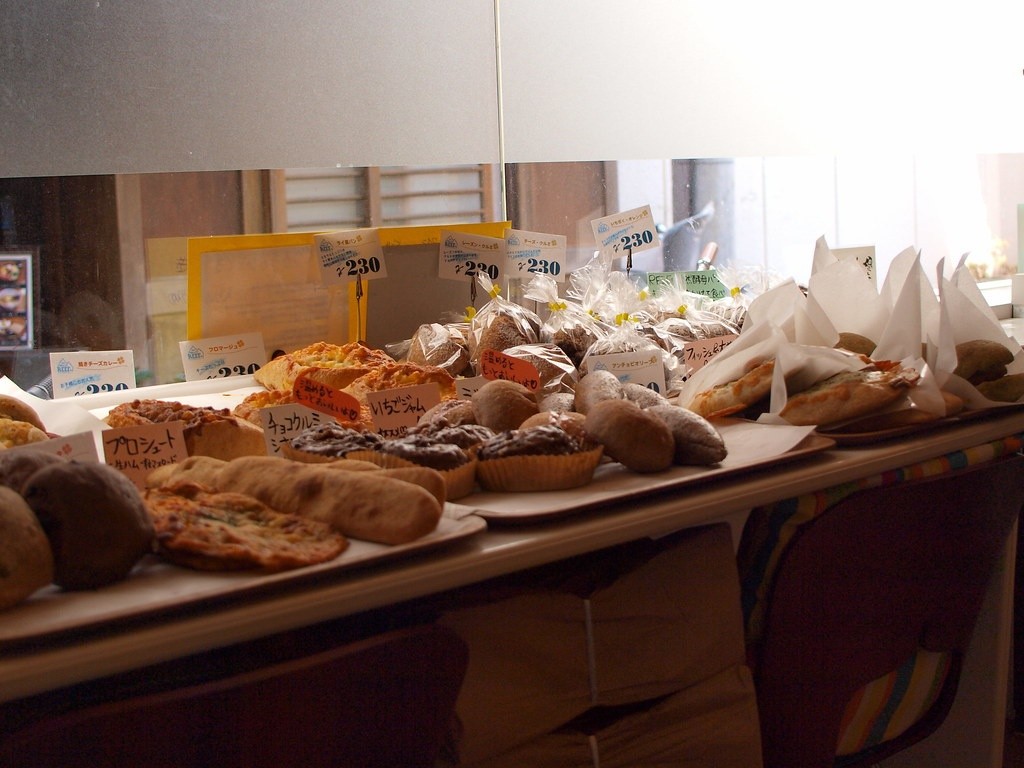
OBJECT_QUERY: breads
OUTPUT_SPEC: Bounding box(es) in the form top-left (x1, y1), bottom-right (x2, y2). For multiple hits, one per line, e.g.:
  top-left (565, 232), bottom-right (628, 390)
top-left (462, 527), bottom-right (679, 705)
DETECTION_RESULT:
top-left (0, 309), bottom-right (1024, 613)
